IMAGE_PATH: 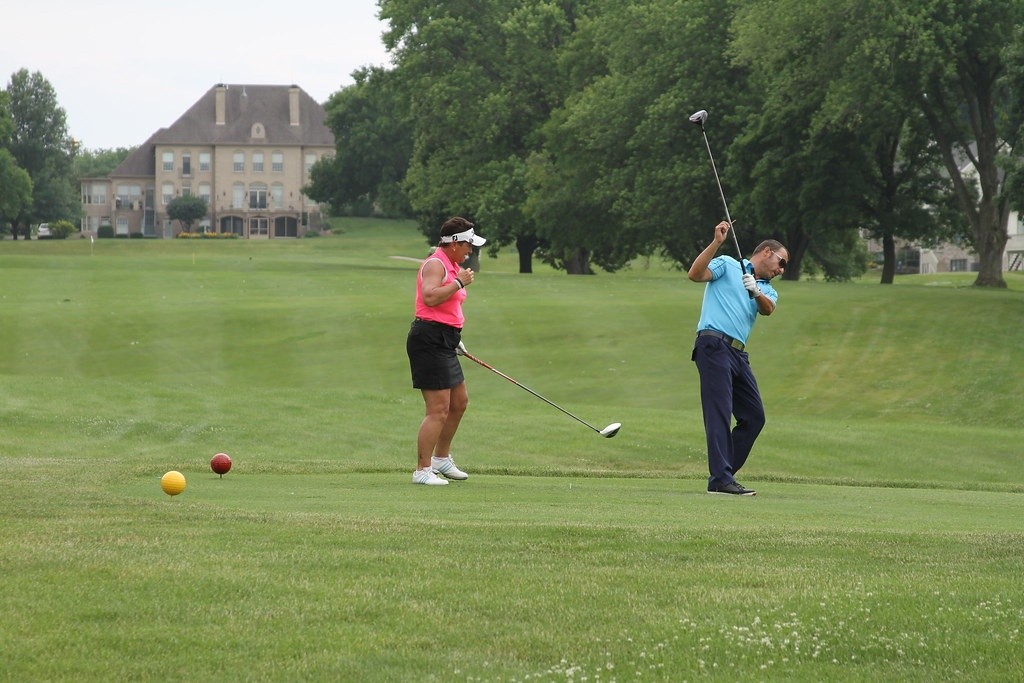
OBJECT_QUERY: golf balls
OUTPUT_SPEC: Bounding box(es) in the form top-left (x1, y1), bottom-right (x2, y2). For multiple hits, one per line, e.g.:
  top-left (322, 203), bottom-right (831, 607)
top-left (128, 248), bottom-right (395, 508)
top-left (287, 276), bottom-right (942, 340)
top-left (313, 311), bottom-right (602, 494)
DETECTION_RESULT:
top-left (210, 453), bottom-right (234, 474)
top-left (160, 470), bottom-right (186, 496)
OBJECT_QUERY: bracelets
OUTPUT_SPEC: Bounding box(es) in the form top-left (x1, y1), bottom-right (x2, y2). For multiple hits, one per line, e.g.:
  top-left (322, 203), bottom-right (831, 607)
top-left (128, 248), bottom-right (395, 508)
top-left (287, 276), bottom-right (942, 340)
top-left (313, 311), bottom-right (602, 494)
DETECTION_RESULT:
top-left (455, 277), bottom-right (464, 289)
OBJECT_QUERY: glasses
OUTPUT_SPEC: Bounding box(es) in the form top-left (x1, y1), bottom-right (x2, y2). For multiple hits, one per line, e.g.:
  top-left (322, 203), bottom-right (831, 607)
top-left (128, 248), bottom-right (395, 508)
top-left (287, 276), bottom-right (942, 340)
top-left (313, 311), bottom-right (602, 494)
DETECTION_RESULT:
top-left (771, 250), bottom-right (788, 271)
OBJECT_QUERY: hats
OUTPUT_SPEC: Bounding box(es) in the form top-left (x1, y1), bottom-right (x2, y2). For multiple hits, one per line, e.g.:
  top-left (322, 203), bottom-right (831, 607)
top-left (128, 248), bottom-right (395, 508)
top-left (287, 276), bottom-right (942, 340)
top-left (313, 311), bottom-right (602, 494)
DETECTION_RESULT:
top-left (441, 227), bottom-right (487, 247)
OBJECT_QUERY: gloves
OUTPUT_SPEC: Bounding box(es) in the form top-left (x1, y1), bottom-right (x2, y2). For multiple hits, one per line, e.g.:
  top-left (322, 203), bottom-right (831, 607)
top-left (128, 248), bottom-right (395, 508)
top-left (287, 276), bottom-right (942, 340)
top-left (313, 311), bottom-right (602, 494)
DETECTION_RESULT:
top-left (455, 340), bottom-right (467, 356)
top-left (742, 273), bottom-right (762, 297)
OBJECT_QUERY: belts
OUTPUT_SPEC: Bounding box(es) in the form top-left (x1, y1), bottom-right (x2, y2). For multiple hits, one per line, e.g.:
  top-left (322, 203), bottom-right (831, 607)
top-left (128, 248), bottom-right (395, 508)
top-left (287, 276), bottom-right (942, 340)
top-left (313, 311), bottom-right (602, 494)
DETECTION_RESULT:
top-left (697, 329), bottom-right (745, 351)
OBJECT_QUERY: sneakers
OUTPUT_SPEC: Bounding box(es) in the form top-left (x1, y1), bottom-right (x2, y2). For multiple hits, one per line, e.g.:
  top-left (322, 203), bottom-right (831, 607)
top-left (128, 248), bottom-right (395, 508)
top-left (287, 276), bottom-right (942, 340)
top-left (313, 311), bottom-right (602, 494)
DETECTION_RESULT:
top-left (431, 453), bottom-right (468, 480)
top-left (413, 466), bottom-right (449, 485)
top-left (707, 480), bottom-right (757, 496)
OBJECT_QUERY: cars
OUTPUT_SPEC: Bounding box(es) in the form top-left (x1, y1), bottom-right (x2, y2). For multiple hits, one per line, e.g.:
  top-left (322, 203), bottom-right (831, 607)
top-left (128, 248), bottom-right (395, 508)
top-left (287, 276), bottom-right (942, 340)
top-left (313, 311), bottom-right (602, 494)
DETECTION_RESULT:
top-left (37, 223), bottom-right (53, 239)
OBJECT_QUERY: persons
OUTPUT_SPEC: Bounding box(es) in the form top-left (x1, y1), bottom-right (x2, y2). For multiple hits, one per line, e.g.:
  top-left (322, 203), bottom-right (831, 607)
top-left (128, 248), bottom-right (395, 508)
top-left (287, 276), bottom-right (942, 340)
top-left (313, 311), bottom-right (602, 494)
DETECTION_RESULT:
top-left (688, 221), bottom-right (791, 496)
top-left (406, 217), bottom-right (486, 485)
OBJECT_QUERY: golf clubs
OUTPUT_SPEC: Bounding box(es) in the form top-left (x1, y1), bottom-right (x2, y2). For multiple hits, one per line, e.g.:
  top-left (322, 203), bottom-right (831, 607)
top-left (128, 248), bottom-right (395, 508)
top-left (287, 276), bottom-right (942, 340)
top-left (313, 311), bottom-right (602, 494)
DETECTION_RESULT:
top-left (689, 109), bottom-right (754, 300)
top-left (457, 346), bottom-right (622, 438)
